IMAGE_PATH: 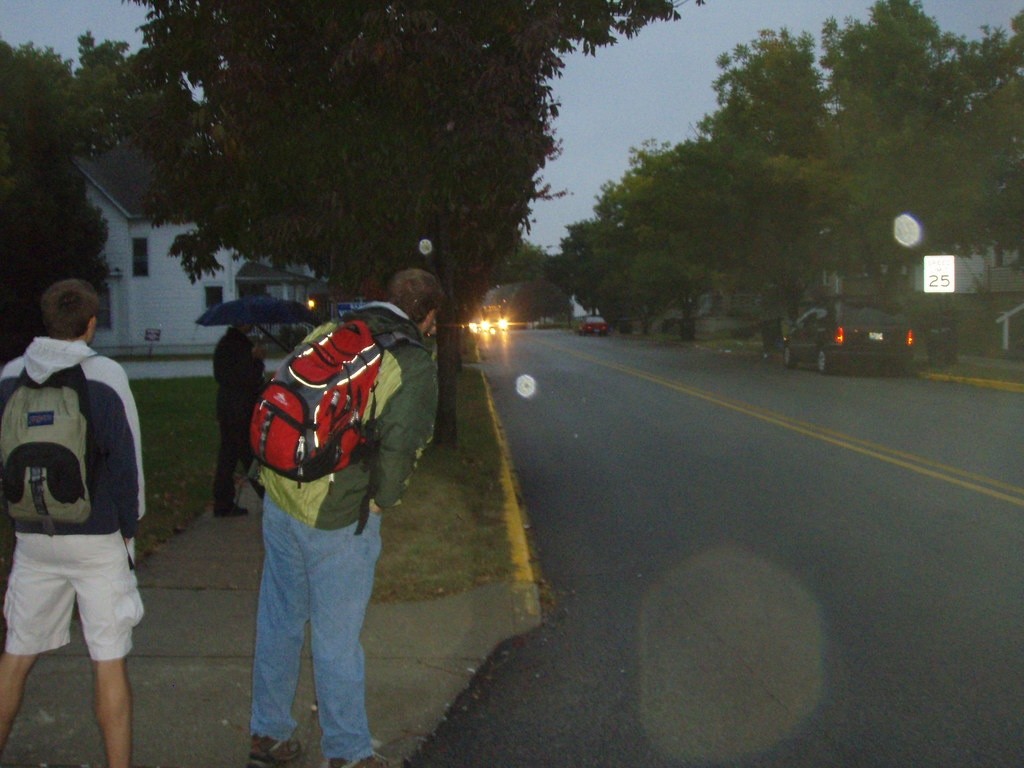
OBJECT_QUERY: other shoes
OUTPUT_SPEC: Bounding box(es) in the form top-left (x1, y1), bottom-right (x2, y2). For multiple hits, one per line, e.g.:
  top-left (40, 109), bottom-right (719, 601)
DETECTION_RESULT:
top-left (213, 496), bottom-right (248, 518)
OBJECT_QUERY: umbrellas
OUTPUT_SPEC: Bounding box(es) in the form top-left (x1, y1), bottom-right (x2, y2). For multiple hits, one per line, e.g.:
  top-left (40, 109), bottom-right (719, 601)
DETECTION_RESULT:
top-left (195, 296), bottom-right (323, 326)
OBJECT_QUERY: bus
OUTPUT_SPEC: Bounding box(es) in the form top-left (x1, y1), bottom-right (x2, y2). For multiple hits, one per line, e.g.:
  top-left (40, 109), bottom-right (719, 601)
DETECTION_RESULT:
top-left (481, 307), bottom-right (505, 334)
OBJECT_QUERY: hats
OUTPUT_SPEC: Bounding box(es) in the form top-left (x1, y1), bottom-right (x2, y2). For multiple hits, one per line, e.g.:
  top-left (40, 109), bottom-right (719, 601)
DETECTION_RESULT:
top-left (390, 269), bottom-right (445, 297)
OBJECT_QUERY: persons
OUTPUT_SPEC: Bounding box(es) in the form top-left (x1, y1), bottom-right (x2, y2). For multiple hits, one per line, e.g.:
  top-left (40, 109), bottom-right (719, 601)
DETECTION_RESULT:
top-left (248, 269), bottom-right (446, 768)
top-left (0, 278), bottom-right (146, 768)
top-left (213, 324), bottom-right (265, 517)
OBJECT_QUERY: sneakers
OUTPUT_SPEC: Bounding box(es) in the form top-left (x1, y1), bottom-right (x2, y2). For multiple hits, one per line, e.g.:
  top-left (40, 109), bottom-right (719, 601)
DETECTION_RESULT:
top-left (327, 751), bottom-right (400, 768)
top-left (246, 736), bottom-right (306, 768)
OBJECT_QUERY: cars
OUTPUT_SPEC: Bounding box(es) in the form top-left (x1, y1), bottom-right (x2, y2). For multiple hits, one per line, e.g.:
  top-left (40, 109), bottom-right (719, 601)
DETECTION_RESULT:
top-left (578, 315), bottom-right (608, 337)
top-left (782, 295), bottom-right (914, 373)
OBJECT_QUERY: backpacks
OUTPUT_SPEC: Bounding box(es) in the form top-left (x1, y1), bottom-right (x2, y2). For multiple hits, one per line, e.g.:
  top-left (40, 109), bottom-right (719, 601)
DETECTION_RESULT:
top-left (0, 363), bottom-right (97, 536)
top-left (247, 306), bottom-right (433, 537)
